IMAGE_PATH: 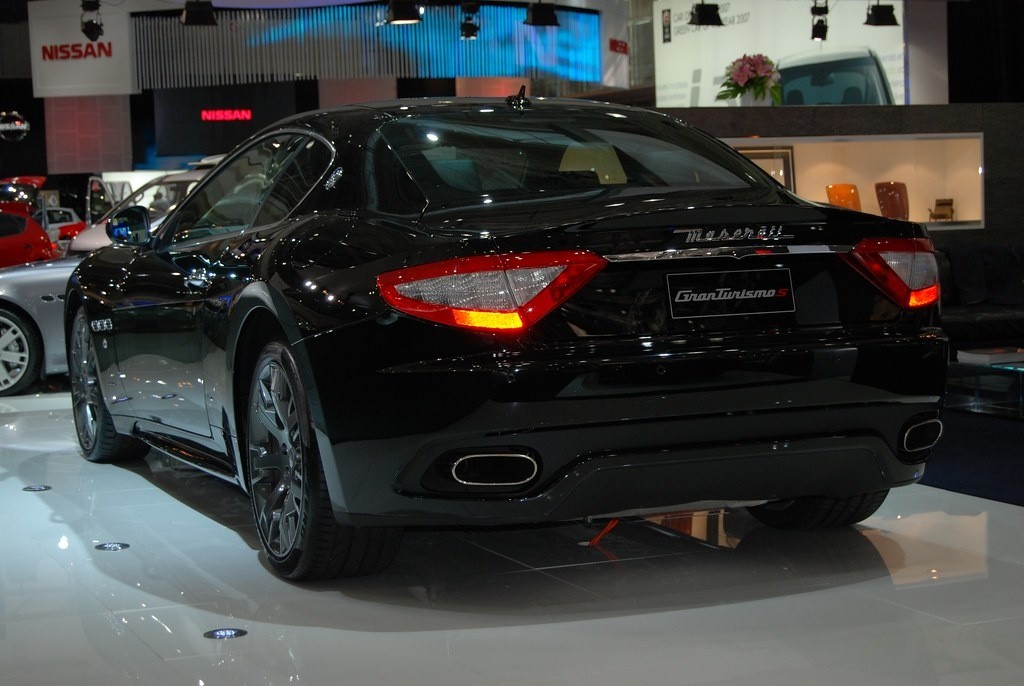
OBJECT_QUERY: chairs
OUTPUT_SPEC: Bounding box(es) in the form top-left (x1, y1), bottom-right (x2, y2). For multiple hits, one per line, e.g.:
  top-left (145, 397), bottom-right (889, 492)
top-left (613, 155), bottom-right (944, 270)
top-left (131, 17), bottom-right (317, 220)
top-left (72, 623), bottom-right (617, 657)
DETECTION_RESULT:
top-left (927, 198), bottom-right (954, 222)
top-left (841, 87), bottom-right (863, 104)
top-left (787, 90), bottom-right (805, 105)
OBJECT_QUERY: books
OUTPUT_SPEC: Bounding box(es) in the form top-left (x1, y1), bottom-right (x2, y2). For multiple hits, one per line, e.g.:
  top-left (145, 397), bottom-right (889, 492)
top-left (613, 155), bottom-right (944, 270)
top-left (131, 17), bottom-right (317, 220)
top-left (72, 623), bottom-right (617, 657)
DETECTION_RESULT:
top-left (957, 347), bottom-right (1024, 364)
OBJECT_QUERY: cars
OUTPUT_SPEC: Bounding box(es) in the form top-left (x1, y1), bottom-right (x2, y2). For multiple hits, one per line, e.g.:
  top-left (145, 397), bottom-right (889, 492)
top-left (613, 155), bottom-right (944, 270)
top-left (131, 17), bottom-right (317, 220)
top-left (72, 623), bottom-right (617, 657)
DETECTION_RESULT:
top-left (62, 97), bottom-right (955, 584)
top-left (0, 194), bottom-right (262, 399)
top-left (0, 168), bottom-right (146, 270)
top-left (68, 154), bottom-right (230, 256)
top-left (768, 46), bottom-right (897, 106)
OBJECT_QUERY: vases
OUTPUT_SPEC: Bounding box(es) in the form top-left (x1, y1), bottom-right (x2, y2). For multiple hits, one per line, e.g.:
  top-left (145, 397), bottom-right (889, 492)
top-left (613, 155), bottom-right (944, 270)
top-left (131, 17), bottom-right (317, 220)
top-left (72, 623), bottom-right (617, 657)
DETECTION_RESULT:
top-left (741, 88), bottom-right (771, 106)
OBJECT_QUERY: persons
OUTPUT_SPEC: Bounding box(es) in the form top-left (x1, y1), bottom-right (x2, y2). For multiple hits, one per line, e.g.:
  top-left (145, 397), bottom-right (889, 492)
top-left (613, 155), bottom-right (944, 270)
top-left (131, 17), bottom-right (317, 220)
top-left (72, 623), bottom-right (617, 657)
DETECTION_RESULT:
top-left (151, 193), bottom-right (167, 212)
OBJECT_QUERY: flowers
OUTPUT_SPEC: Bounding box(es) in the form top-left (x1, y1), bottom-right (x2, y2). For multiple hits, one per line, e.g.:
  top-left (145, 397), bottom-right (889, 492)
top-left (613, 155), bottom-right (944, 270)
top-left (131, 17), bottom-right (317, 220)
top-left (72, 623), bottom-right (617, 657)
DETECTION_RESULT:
top-left (716, 53), bottom-right (783, 107)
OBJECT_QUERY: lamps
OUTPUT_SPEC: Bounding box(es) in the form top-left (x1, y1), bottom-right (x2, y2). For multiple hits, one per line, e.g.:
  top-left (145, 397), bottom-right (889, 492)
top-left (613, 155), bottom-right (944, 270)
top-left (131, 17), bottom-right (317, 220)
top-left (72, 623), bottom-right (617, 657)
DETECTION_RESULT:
top-left (457, 3), bottom-right (481, 41)
top-left (685, 0), bottom-right (725, 27)
top-left (178, 0), bottom-right (219, 26)
top-left (863, 0), bottom-right (901, 27)
top-left (810, 0), bottom-right (830, 42)
top-left (78, 0), bottom-right (105, 41)
top-left (523, 0), bottom-right (561, 27)
top-left (384, 1), bottom-right (425, 25)
